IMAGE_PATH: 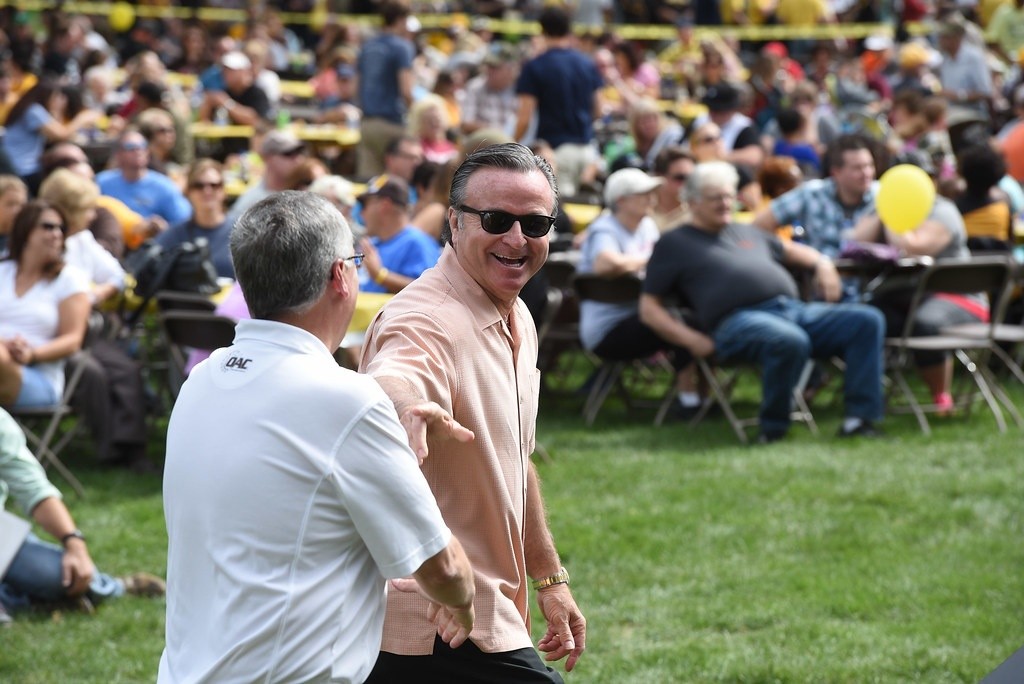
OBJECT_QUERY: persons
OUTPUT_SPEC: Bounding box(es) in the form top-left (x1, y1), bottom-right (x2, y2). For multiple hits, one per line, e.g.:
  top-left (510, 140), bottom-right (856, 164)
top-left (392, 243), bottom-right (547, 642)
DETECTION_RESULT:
top-left (0, 203), bottom-right (90, 412)
top-left (844, 151), bottom-right (991, 417)
top-left (638, 160), bottom-right (884, 443)
top-left (37, 167), bottom-right (149, 472)
top-left (356, 142), bottom-right (585, 684)
top-left (2, 0), bottom-right (1024, 295)
top-left (1, 405), bottom-right (166, 621)
top-left (751, 135), bottom-right (881, 303)
top-left (156, 190), bottom-right (475, 684)
top-left (575, 166), bottom-right (727, 422)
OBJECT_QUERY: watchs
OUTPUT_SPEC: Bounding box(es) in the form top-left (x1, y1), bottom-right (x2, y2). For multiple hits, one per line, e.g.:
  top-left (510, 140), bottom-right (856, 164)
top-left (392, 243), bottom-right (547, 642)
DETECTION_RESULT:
top-left (532, 566), bottom-right (570, 590)
top-left (62, 530), bottom-right (85, 547)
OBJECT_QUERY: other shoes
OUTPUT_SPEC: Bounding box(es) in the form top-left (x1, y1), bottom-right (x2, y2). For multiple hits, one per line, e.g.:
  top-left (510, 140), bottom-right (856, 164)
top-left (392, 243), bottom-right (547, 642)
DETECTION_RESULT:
top-left (122, 572), bottom-right (165, 598)
top-left (672, 397), bottom-right (711, 417)
top-left (837, 422), bottom-right (880, 438)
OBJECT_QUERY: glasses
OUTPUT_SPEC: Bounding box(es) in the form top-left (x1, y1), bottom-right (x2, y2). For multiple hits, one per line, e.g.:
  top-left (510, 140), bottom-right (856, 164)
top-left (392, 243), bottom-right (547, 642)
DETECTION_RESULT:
top-left (459, 204), bottom-right (557, 239)
top-left (331, 253), bottom-right (365, 280)
top-left (38, 222), bottom-right (64, 233)
top-left (281, 145), bottom-right (305, 157)
top-left (191, 182), bottom-right (222, 191)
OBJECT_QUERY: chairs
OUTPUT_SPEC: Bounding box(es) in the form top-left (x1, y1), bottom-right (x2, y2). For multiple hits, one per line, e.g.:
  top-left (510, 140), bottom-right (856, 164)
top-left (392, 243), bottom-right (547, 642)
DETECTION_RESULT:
top-left (5, 245), bottom-right (235, 506)
top-left (539, 232), bottom-right (1024, 440)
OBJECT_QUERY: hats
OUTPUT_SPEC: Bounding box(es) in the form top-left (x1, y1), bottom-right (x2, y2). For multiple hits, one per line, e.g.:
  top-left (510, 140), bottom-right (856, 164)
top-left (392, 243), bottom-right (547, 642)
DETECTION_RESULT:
top-left (603, 168), bottom-right (665, 203)
top-left (358, 173), bottom-right (411, 204)
top-left (222, 51), bottom-right (252, 71)
